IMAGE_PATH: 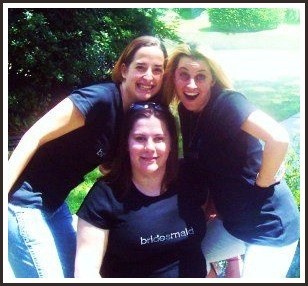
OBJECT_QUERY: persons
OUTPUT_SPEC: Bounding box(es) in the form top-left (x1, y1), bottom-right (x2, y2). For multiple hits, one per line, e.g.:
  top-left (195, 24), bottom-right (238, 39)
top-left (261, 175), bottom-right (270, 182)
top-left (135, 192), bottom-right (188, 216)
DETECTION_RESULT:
top-left (165, 41), bottom-right (300, 278)
top-left (8, 33), bottom-right (172, 279)
top-left (71, 101), bottom-right (212, 278)
